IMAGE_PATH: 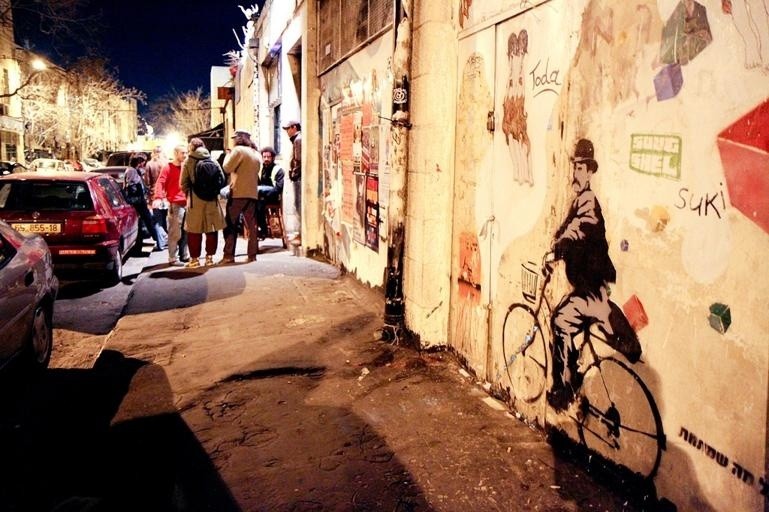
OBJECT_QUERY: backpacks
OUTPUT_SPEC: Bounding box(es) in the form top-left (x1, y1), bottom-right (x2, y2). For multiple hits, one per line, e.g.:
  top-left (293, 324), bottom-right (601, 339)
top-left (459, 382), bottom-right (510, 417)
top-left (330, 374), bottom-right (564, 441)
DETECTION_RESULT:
top-left (186, 154), bottom-right (223, 201)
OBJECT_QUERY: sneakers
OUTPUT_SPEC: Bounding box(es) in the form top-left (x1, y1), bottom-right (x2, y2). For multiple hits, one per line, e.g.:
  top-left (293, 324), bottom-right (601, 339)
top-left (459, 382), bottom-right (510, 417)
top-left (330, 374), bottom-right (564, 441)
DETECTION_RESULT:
top-left (545, 367), bottom-right (585, 408)
top-left (180, 256), bottom-right (190, 262)
top-left (287, 232), bottom-right (303, 247)
top-left (247, 254), bottom-right (257, 262)
top-left (169, 260), bottom-right (185, 267)
top-left (216, 256), bottom-right (233, 265)
top-left (185, 257), bottom-right (201, 268)
top-left (204, 254), bottom-right (213, 267)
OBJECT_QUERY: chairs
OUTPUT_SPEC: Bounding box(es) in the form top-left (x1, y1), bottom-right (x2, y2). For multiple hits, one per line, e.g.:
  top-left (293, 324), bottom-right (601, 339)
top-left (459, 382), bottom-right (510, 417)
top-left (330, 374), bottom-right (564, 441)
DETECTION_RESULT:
top-left (257, 179), bottom-right (289, 253)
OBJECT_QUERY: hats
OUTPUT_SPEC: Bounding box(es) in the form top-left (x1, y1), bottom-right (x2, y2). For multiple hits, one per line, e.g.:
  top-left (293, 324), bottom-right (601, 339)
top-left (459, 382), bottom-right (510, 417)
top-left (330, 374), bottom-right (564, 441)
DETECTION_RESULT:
top-left (188, 137), bottom-right (205, 153)
top-left (230, 129), bottom-right (252, 139)
top-left (567, 136), bottom-right (602, 178)
top-left (281, 120), bottom-right (302, 130)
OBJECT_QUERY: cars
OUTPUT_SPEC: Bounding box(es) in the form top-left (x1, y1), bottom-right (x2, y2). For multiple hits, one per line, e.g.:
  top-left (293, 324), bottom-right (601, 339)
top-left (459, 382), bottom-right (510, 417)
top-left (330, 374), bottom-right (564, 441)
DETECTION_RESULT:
top-left (1, 150), bottom-right (160, 369)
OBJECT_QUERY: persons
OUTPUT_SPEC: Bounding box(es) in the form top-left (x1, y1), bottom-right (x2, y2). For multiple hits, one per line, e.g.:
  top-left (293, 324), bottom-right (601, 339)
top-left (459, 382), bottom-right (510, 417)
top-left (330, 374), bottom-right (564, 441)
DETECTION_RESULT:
top-left (122, 119), bottom-right (302, 269)
top-left (540, 141), bottom-right (616, 409)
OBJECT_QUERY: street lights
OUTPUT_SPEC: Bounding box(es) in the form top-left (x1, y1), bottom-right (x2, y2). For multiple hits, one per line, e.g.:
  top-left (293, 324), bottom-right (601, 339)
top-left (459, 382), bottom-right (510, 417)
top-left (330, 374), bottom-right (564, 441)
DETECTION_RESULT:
top-left (29, 57), bottom-right (50, 146)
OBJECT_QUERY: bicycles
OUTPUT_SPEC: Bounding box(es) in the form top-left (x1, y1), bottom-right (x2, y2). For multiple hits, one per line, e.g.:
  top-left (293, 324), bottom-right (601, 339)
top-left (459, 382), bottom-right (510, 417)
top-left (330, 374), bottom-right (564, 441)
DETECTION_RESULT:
top-left (501, 247), bottom-right (668, 484)
